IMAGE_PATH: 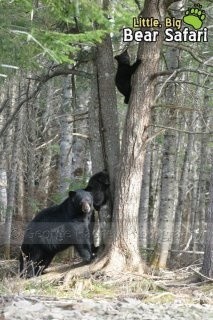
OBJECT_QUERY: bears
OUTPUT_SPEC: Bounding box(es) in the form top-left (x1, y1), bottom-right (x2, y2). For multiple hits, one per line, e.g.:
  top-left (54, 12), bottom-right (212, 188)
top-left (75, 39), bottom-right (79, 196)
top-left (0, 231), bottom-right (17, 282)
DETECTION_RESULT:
top-left (114, 48), bottom-right (142, 105)
top-left (18, 170), bottom-right (110, 279)
